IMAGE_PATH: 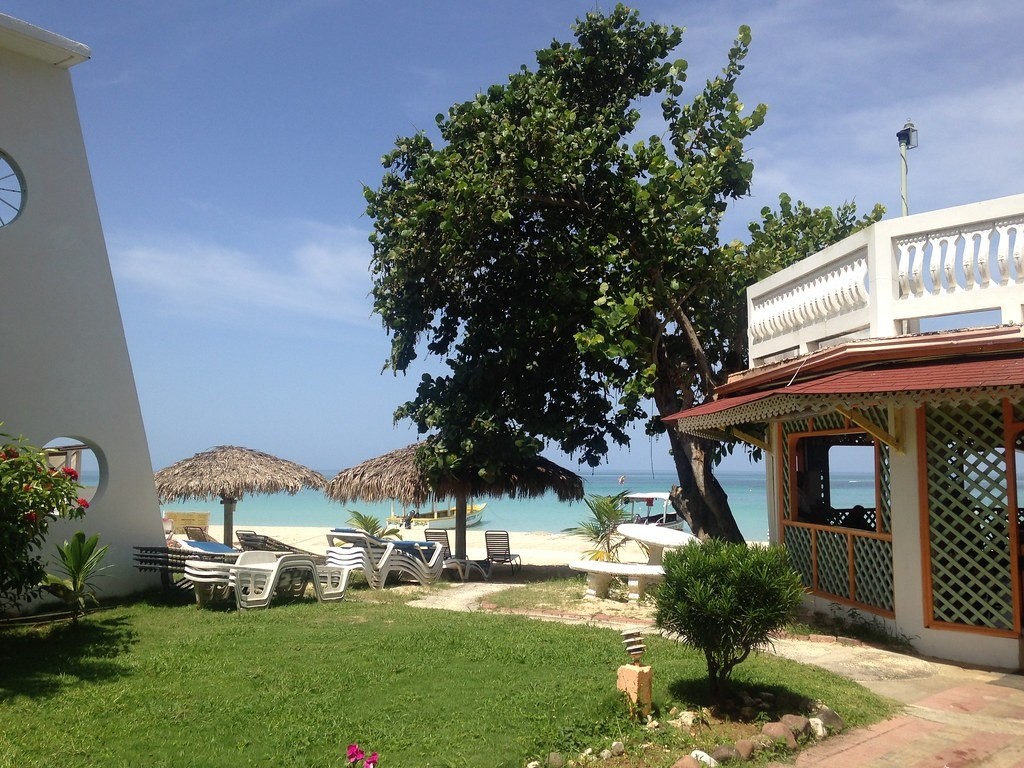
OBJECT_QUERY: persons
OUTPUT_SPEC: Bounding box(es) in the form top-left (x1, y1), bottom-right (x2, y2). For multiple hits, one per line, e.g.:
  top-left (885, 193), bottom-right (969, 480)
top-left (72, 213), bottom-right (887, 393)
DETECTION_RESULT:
top-left (797, 470), bottom-right (832, 526)
top-left (840, 505), bottom-right (874, 531)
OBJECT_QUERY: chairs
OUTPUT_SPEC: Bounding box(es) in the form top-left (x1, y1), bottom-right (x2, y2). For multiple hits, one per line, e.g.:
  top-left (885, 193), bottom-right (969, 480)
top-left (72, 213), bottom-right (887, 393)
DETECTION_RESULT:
top-left (133, 524), bottom-right (522, 611)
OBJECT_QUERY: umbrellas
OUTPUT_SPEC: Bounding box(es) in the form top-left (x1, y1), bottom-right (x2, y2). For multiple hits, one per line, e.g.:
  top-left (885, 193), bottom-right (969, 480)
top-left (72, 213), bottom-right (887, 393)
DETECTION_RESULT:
top-left (152, 445), bottom-right (329, 551)
top-left (323, 438), bottom-right (585, 560)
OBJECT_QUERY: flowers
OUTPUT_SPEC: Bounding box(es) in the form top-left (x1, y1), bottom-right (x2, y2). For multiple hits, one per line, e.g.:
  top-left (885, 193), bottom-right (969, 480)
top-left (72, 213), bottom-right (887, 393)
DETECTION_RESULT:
top-left (347, 743), bottom-right (379, 768)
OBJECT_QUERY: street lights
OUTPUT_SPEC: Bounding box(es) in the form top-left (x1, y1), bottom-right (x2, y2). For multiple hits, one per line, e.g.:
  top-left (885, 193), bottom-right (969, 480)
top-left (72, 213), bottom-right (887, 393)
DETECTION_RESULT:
top-left (896, 117), bottom-right (919, 218)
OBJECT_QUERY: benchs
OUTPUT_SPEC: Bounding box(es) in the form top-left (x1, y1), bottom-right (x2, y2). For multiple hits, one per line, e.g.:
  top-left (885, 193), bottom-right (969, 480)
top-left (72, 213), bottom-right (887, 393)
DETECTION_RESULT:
top-left (570, 560), bottom-right (668, 604)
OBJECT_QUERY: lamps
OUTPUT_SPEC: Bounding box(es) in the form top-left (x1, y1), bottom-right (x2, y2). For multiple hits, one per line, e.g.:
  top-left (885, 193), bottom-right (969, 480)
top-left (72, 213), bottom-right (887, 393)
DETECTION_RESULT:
top-left (618, 624), bottom-right (655, 716)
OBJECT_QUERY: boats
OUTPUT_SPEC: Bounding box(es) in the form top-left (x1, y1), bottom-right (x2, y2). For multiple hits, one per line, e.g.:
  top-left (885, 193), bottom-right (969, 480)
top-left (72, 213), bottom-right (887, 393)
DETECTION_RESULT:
top-left (387, 490), bottom-right (487, 528)
top-left (598, 491), bottom-right (684, 531)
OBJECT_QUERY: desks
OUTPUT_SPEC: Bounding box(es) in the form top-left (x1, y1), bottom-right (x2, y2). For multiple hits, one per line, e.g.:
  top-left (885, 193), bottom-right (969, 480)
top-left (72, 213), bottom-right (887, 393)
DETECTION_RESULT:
top-left (618, 523), bottom-right (701, 566)
